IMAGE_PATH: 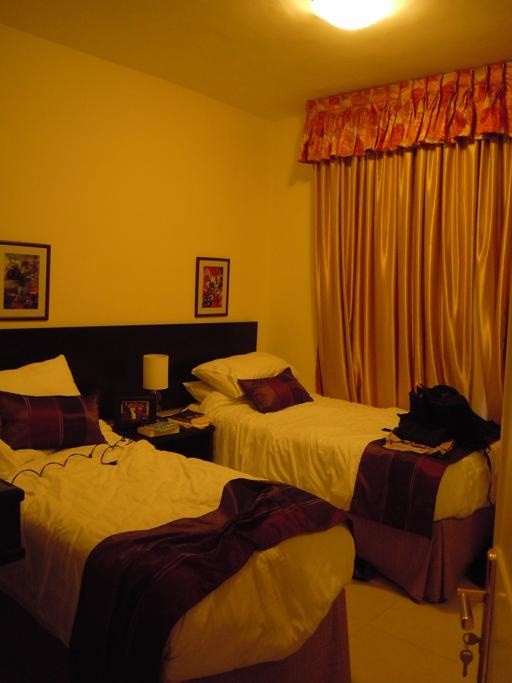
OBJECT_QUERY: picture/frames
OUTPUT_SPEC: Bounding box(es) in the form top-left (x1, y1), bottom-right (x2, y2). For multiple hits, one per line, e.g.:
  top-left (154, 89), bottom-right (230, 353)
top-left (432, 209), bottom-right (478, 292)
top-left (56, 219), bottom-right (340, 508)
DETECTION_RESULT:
top-left (111, 391), bottom-right (158, 425)
top-left (0, 239), bottom-right (53, 324)
top-left (193, 253), bottom-right (232, 319)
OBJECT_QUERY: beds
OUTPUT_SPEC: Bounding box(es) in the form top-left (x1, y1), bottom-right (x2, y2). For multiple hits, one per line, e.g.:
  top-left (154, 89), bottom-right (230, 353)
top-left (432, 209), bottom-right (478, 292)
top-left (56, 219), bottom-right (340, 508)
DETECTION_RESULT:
top-left (0, 413), bottom-right (364, 682)
top-left (169, 394), bottom-right (502, 607)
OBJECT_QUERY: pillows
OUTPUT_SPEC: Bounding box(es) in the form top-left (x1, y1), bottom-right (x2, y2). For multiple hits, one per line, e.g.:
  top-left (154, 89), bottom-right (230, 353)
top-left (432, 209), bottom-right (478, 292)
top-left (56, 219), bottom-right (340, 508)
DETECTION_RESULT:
top-left (181, 378), bottom-right (215, 401)
top-left (187, 350), bottom-right (300, 400)
top-left (0, 350), bottom-right (85, 396)
top-left (0, 387), bottom-right (112, 453)
top-left (235, 363), bottom-right (316, 414)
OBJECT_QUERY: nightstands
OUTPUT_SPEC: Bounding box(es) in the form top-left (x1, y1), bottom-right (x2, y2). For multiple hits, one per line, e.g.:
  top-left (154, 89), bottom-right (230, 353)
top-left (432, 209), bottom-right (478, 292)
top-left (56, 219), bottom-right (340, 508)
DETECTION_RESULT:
top-left (1, 479), bottom-right (25, 568)
top-left (110, 410), bottom-right (216, 462)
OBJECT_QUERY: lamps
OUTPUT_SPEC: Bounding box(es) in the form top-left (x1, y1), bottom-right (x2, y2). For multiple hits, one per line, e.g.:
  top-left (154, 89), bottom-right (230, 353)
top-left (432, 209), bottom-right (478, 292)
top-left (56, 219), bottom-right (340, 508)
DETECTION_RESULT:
top-left (140, 351), bottom-right (173, 420)
